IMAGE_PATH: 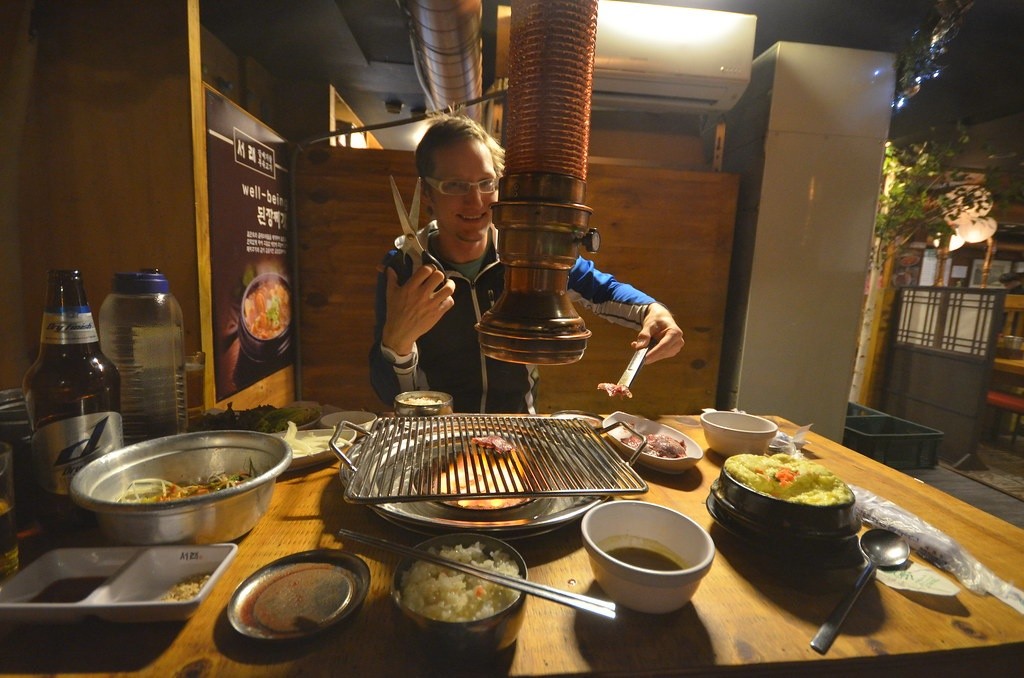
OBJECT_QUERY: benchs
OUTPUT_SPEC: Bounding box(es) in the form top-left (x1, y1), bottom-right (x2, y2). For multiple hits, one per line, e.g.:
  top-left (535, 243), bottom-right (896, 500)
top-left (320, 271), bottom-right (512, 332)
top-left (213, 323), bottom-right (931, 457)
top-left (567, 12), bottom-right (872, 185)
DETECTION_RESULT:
top-left (986, 390), bottom-right (1024, 415)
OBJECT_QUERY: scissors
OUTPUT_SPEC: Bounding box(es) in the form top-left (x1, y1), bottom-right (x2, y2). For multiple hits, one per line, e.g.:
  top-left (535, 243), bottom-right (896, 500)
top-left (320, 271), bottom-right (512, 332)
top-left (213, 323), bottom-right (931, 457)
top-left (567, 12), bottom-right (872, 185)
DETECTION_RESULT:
top-left (388, 173), bottom-right (449, 287)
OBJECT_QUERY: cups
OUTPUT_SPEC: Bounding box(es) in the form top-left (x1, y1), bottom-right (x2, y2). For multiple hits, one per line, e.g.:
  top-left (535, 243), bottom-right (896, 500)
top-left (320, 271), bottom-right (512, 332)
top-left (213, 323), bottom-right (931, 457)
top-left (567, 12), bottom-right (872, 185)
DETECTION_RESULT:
top-left (0, 443), bottom-right (19, 589)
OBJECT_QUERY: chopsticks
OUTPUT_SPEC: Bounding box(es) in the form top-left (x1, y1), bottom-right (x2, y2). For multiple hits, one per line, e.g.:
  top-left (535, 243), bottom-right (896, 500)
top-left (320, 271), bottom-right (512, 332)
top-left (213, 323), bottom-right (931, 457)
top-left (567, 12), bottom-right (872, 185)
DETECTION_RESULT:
top-left (337, 527), bottom-right (616, 622)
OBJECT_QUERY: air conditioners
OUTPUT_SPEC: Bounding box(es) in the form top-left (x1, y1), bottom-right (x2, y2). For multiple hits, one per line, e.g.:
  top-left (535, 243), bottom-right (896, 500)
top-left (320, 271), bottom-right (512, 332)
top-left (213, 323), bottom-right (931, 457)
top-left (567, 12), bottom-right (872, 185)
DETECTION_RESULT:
top-left (591, 0), bottom-right (758, 114)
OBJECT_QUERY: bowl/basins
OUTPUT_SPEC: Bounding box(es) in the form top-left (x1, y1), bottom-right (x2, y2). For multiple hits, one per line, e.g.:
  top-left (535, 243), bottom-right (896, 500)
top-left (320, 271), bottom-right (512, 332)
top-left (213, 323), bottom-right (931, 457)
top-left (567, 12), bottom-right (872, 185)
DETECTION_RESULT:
top-left (322, 410), bottom-right (377, 435)
top-left (582, 500), bottom-right (714, 615)
top-left (701, 412), bottom-right (782, 461)
top-left (391, 533), bottom-right (532, 659)
top-left (396, 389), bottom-right (454, 420)
top-left (239, 273), bottom-right (294, 365)
top-left (74, 431), bottom-right (292, 544)
top-left (708, 450), bottom-right (864, 555)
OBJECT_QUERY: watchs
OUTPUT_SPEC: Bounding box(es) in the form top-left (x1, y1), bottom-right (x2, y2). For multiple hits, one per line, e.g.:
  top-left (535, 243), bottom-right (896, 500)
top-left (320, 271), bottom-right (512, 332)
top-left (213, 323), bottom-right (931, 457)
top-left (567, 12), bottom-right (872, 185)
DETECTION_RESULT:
top-left (380, 340), bottom-right (412, 364)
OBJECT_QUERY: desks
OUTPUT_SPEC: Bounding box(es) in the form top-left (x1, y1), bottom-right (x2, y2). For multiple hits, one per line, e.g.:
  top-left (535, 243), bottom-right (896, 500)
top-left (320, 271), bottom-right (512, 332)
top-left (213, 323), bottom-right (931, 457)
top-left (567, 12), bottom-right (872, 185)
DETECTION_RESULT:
top-left (982, 354), bottom-right (1024, 445)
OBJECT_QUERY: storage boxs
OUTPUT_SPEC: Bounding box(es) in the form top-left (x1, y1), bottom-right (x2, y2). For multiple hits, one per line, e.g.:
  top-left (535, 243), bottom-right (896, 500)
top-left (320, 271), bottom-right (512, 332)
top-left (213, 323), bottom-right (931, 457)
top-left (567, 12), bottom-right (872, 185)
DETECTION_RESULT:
top-left (846, 400), bottom-right (890, 417)
top-left (842, 416), bottom-right (944, 470)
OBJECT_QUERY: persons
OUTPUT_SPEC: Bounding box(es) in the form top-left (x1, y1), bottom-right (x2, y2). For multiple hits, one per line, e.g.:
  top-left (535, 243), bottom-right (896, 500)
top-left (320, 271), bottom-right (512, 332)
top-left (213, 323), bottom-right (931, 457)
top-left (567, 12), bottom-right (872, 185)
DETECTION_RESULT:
top-left (369, 101), bottom-right (685, 414)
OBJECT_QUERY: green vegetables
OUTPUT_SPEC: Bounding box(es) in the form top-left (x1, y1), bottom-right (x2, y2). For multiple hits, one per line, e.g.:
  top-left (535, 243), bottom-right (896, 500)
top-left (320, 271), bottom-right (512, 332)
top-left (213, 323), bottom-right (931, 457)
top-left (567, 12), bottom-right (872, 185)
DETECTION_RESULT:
top-left (185, 403), bottom-right (317, 435)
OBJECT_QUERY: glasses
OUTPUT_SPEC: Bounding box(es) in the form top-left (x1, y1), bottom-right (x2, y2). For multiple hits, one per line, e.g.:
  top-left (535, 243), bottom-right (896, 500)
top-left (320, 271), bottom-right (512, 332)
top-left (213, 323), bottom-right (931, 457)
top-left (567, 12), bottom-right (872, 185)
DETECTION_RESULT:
top-left (422, 175), bottom-right (499, 197)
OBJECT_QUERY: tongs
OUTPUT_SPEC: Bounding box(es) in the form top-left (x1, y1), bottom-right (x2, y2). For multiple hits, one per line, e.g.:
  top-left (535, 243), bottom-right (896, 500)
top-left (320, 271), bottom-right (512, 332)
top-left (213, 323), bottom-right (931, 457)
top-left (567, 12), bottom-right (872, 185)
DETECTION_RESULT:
top-left (616, 345), bottom-right (650, 390)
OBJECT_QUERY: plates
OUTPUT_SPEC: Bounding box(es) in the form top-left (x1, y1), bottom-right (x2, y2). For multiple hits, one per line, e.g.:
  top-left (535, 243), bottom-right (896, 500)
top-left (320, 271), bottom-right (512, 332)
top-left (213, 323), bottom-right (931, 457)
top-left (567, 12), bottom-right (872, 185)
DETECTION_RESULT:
top-left (269, 428), bottom-right (356, 470)
top-left (603, 411), bottom-right (703, 473)
top-left (551, 411), bottom-right (605, 432)
top-left (225, 549), bottom-right (371, 645)
top-left (0, 543), bottom-right (237, 625)
top-left (269, 410), bottom-right (321, 432)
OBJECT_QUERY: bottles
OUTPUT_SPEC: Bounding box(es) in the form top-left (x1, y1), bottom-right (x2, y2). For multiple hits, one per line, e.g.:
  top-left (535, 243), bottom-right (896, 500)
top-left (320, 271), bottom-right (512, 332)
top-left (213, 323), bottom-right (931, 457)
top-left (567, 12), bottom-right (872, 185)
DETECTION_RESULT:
top-left (22, 269), bottom-right (124, 524)
top-left (101, 273), bottom-right (187, 443)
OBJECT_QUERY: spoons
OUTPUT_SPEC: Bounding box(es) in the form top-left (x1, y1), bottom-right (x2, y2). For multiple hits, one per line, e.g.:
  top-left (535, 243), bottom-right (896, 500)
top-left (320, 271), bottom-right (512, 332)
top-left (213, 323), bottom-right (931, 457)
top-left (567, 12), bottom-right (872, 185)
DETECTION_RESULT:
top-left (810, 528), bottom-right (911, 654)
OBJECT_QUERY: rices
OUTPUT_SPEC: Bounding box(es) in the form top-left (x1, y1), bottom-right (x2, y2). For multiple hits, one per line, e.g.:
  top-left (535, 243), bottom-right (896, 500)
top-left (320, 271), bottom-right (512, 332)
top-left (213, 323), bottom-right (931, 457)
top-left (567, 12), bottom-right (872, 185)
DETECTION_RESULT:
top-left (398, 541), bottom-right (522, 623)
top-left (398, 396), bottom-right (446, 405)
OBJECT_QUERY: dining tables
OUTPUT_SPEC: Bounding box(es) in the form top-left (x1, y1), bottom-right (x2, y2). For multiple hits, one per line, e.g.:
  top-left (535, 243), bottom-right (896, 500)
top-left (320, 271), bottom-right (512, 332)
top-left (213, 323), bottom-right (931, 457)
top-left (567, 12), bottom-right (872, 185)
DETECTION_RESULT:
top-left (0, 415), bottom-right (1024, 678)
